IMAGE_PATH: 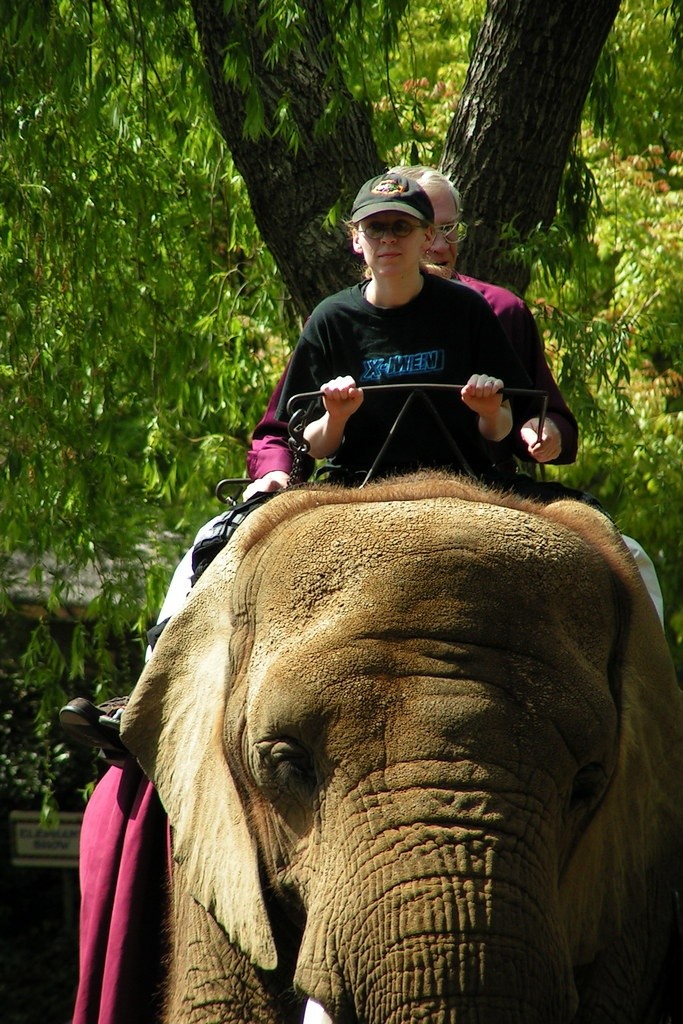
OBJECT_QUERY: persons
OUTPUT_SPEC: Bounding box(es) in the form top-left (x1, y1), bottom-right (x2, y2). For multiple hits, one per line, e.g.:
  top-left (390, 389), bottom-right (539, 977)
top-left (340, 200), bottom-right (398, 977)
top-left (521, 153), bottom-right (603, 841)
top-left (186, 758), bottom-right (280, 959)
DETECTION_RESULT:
top-left (62, 166), bottom-right (666, 760)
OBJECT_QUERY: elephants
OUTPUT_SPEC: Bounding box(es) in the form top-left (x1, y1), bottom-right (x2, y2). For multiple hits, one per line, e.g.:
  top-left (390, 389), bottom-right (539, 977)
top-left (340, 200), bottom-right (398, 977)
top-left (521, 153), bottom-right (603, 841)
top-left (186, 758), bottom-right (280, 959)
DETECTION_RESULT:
top-left (120, 468), bottom-right (683, 1024)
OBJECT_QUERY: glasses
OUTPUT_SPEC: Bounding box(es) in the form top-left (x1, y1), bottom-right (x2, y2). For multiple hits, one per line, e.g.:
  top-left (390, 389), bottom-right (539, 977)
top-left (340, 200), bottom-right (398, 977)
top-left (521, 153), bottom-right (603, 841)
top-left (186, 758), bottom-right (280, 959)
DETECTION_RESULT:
top-left (358, 218), bottom-right (424, 238)
top-left (437, 222), bottom-right (469, 244)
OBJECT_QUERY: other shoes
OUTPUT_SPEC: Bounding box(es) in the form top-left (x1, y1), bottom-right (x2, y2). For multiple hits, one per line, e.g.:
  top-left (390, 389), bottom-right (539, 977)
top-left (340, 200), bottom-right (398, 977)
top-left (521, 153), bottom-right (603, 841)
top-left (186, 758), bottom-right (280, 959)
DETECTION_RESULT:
top-left (100, 707), bottom-right (123, 729)
top-left (59, 696), bottom-right (130, 760)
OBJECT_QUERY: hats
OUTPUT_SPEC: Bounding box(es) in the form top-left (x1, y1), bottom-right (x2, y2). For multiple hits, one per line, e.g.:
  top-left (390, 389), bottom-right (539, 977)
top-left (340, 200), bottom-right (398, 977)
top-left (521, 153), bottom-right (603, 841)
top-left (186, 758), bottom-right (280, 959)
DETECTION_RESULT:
top-left (350, 174), bottom-right (435, 227)
